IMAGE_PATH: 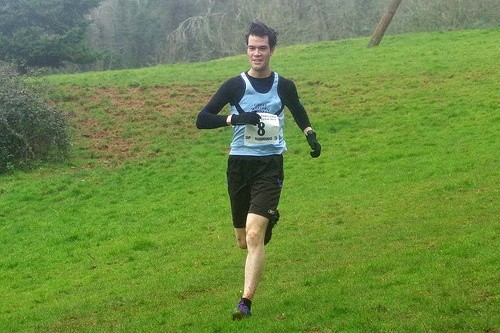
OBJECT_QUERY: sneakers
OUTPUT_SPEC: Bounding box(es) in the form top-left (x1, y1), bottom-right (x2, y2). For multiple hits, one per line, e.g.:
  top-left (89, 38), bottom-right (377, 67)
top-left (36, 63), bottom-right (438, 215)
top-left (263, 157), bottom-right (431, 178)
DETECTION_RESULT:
top-left (232, 296), bottom-right (252, 320)
top-left (261, 210), bottom-right (280, 245)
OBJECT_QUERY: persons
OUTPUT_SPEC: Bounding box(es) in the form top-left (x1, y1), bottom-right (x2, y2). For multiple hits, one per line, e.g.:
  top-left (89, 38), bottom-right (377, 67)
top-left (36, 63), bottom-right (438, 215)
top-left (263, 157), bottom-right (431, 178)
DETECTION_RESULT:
top-left (195, 21), bottom-right (321, 319)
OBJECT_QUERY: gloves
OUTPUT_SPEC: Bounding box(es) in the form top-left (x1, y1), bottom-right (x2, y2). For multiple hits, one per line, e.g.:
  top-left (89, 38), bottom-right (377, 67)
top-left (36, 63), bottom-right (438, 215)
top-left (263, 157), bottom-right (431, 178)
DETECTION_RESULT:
top-left (305, 130), bottom-right (321, 157)
top-left (231, 110), bottom-right (261, 126)
